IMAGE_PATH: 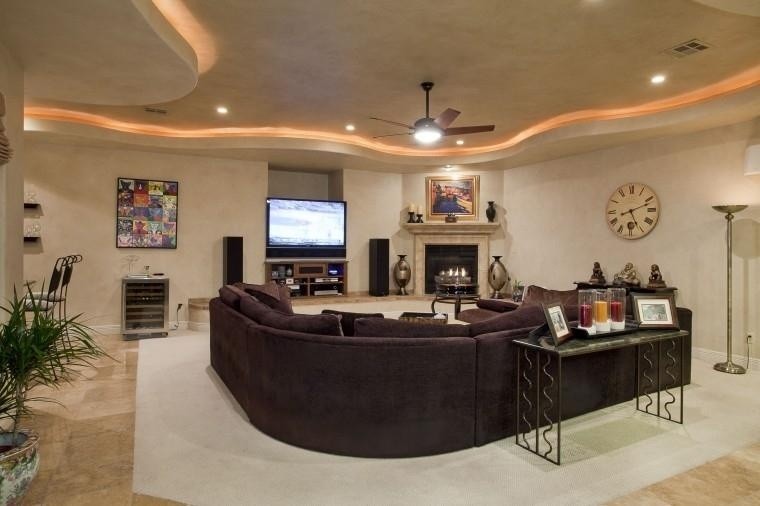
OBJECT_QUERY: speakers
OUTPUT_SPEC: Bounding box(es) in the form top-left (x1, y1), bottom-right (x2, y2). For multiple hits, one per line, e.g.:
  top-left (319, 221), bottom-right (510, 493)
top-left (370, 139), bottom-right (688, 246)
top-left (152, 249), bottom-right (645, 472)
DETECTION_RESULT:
top-left (369, 237), bottom-right (391, 296)
top-left (223, 235), bottom-right (243, 287)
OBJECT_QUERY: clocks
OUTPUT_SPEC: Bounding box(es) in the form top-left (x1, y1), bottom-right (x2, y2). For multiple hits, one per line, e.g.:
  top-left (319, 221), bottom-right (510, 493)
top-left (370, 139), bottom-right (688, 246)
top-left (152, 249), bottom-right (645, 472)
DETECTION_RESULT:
top-left (605, 183), bottom-right (659, 241)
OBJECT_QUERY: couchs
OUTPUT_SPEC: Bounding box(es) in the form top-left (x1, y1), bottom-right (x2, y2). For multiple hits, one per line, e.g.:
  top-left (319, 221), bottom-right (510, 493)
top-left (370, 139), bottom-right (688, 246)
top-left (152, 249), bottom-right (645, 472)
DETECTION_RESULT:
top-left (208, 282), bottom-right (692, 460)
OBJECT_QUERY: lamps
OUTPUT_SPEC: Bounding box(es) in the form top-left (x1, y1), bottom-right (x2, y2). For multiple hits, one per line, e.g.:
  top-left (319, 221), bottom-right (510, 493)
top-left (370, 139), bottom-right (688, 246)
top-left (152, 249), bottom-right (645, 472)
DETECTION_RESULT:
top-left (712, 204), bottom-right (748, 374)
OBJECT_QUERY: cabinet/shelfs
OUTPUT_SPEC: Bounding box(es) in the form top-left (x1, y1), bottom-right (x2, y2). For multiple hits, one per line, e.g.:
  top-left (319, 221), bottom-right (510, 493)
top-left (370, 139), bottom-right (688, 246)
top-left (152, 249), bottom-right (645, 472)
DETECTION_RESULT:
top-left (264, 258), bottom-right (347, 297)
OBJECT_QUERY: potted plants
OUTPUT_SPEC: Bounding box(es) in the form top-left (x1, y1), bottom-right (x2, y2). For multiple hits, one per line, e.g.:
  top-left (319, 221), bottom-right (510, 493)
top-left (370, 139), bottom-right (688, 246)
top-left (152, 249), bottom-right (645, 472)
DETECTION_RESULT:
top-left (0, 277), bottom-right (122, 506)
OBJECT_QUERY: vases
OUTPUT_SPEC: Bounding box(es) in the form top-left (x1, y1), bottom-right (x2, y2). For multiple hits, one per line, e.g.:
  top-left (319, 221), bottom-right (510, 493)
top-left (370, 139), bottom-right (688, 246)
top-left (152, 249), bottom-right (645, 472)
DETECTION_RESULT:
top-left (489, 255), bottom-right (507, 297)
top-left (486, 200), bottom-right (496, 223)
top-left (394, 255), bottom-right (411, 295)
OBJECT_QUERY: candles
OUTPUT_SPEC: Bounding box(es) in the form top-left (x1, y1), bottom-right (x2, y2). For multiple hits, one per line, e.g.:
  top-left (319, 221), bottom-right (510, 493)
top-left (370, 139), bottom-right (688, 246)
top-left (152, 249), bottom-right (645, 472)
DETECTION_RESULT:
top-left (407, 202), bottom-right (415, 212)
top-left (417, 205), bottom-right (422, 214)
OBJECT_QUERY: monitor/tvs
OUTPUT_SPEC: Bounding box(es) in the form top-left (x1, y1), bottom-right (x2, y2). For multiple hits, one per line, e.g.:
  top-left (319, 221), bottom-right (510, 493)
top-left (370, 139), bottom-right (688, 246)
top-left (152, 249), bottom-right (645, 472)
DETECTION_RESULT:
top-left (265, 196), bottom-right (347, 259)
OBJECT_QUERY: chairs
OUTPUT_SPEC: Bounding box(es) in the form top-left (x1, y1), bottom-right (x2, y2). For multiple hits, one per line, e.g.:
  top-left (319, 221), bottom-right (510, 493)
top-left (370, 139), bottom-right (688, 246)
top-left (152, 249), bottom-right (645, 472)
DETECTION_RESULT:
top-left (23, 254), bottom-right (83, 362)
top-left (23, 256), bottom-right (74, 380)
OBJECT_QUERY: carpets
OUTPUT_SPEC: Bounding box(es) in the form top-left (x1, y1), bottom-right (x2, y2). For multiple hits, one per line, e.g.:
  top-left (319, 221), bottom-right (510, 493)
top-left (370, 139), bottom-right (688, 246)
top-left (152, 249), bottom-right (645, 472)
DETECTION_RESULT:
top-left (132, 300), bottom-right (760, 506)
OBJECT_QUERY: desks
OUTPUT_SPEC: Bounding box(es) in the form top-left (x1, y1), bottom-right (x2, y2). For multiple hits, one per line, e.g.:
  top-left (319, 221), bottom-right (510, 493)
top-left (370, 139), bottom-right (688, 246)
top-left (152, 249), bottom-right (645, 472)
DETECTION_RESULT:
top-left (512, 329), bottom-right (690, 466)
top-left (431, 283), bottom-right (480, 319)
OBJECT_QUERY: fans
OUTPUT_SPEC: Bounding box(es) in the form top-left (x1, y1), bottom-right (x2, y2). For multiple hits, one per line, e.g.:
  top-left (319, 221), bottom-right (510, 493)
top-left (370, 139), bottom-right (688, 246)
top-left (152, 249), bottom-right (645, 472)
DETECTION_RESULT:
top-left (369, 81), bottom-right (495, 139)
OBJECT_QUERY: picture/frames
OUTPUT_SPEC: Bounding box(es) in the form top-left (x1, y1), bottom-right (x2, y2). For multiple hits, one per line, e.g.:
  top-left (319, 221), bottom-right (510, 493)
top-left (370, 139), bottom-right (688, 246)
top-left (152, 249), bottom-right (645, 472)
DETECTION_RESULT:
top-left (529, 300), bottom-right (574, 347)
top-left (424, 175), bottom-right (479, 222)
top-left (630, 292), bottom-right (682, 331)
top-left (116, 178), bottom-right (178, 249)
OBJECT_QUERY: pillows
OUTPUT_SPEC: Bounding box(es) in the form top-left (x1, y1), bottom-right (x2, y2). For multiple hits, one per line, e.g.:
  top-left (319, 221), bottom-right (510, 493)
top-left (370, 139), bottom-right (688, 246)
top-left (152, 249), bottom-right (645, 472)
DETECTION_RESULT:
top-left (476, 298), bottom-right (518, 313)
top-left (400, 312), bottom-right (448, 320)
top-left (321, 310), bottom-right (385, 335)
top-left (245, 287), bottom-right (294, 314)
